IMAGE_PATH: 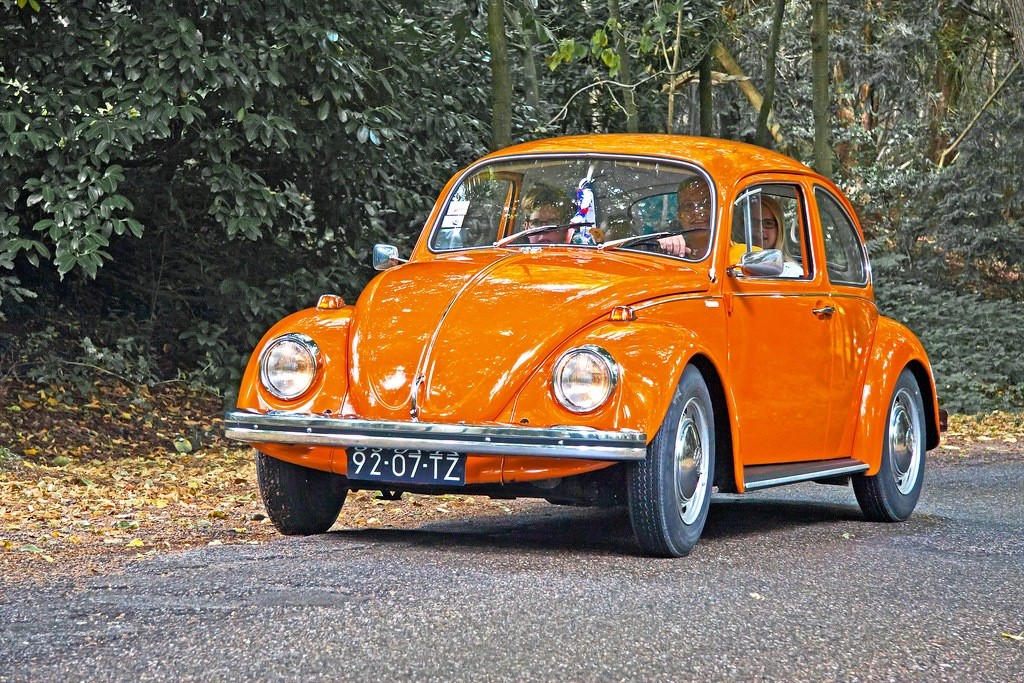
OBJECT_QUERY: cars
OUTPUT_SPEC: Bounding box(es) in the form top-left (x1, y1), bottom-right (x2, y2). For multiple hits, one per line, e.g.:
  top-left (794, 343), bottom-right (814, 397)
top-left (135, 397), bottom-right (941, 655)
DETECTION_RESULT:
top-left (222, 132), bottom-right (948, 558)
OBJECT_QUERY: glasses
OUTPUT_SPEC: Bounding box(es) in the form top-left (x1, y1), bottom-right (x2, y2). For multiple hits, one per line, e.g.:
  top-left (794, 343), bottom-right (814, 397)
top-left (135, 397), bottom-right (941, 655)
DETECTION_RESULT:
top-left (677, 198), bottom-right (711, 212)
top-left (743, 218), bottom-right (778, 229)
top-left (526, 212), bottom-right (568, 230)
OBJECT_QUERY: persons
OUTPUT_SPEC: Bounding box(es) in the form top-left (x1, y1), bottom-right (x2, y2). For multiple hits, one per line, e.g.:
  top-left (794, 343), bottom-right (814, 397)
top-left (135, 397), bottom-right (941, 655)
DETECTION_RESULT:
top-left (740, 195), bottom-right (804, 277)
top-left (648, 176), bottom-right (762, 273)
top-left (521, 186), bottom-right (573, 245)
top-left (599, 215), bottom-right (641, 251)
